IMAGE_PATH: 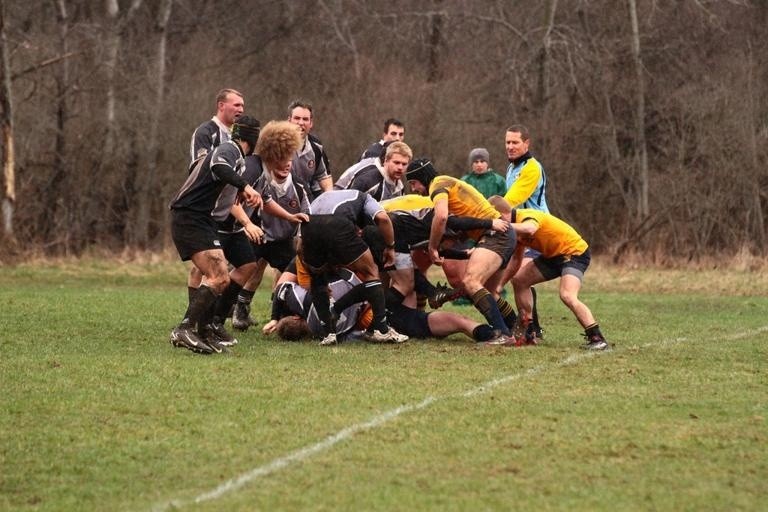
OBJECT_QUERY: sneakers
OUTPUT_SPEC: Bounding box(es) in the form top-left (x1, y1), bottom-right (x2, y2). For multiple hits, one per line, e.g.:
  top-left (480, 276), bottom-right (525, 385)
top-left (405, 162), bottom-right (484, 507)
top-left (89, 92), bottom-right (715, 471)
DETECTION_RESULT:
top-left (512, 310), bottom-right (544, 345)
top-left (328, 306), bottom-right (338, 332)
top-left (208, 328), bottom-right (233, 347)
top-left (484, 334), bottom-right (517, 346)
top-left (230, 304), bottom-right (249, 330)
top-left (317, 333), bottom-right (337, 346)
top-left (245, 305), bottom-right (259, 326)
top-left (428, 284), bottom-right (466, 310)
top-left (475, 328), bottom-right (502, 342)
top-left (197, 326), bottom-right (234, 354)
top-left (579, 339), bottom-right (610, 351)
top-left (169, 326), bottom-right (213, 355)
top-left (365, 323), bottom-right (409, 343)
top-left (213, 321), bottom-right (238, 344)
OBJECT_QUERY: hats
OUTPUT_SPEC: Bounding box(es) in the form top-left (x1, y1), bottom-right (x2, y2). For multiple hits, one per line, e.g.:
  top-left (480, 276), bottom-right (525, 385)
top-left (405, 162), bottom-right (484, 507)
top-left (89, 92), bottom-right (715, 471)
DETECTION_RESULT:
top-left (467, 148), bottom-right (491, 167)
top-left (232, 115), bottom-right (261, 156)
top-left (406, 159), bottom-right (438, 196)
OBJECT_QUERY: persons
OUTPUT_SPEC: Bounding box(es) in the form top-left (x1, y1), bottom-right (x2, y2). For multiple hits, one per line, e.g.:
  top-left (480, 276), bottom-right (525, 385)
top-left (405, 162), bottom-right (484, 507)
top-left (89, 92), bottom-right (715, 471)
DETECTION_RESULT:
top-left (166, 89), bottom-right (607, 350)
